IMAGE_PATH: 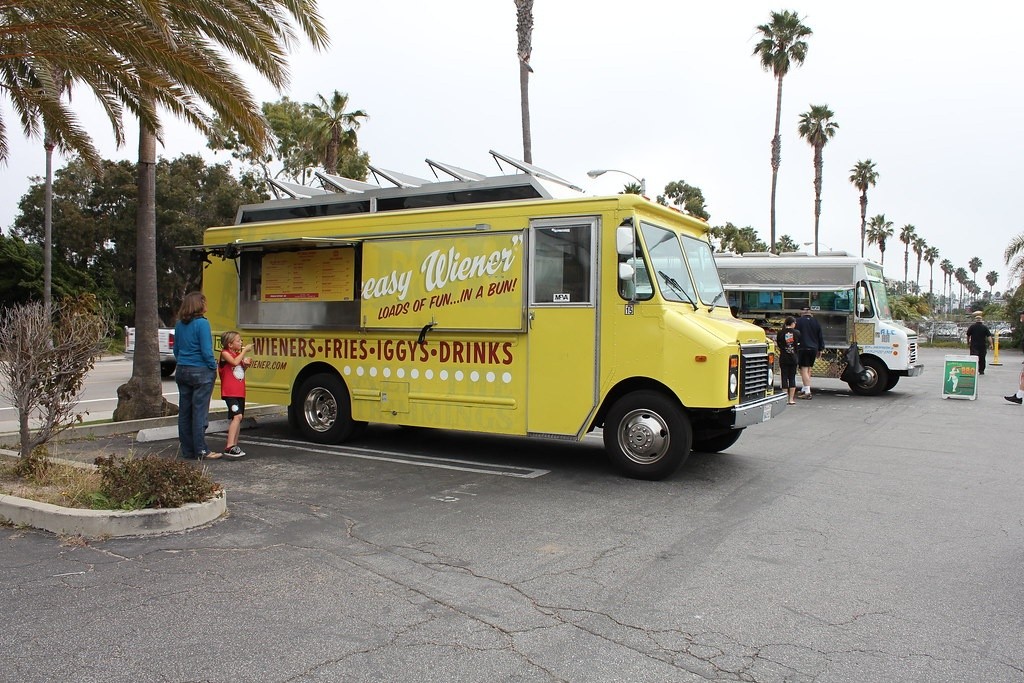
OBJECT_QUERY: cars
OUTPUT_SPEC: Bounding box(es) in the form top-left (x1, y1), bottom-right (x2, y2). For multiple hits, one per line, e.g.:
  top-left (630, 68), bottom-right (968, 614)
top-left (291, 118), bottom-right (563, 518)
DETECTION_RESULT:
top-left (989, 324), bottom-right (1011, 337)
top-left (926, 321), bottom-right (961, 343)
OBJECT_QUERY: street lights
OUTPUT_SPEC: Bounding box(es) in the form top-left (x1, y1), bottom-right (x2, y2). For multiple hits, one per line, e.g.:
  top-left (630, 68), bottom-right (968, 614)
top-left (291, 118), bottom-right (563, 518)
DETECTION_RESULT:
top-left (804, 242), bottom-right (832, 252)
top-left (586, 169), bottom-right (645, 196)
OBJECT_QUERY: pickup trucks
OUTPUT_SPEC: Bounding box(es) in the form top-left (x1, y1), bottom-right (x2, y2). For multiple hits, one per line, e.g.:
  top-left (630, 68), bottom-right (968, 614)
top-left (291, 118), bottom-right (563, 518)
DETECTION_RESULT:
top-left (125, 326), bottom-right (176, 377)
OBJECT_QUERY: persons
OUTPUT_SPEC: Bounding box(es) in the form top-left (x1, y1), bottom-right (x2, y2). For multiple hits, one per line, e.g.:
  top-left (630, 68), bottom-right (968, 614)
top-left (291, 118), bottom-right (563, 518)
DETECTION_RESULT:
top-left (730, 306), bottom-right (739, 318)
top-left (173, 292), bottom-right (222, 459)
top-left (797, 305), bottom-right (825, 399)
top-left (217, 331), bottom-right (254, 456)
top-left (966, 316), bottom-right (994, 375)
top-left (1004, 369), bottom-right (1024, 404)
top-left (777, 316), bottom-right (802, 404)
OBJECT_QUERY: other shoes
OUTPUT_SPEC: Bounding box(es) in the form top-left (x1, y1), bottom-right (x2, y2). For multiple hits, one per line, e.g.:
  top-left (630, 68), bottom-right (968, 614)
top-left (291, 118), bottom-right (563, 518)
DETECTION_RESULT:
top-left (800, 391), bottom-right (812, 400)
top-left (183, 449), bottom-right (223, 461)
top-left (978, 371), bottom-right (985, 375)
top-left (224, 445), bottom-right (246, 456)
top-left (786, 401), bottom-right (796, 405)
top-left (1004, 393), bottom-right (1022, 404)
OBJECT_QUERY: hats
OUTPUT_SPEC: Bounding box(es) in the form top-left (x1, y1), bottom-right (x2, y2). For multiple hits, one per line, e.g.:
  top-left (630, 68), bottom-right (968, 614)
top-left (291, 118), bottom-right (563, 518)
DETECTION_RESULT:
top-left (1019, 310), bottom-right (1024, 314)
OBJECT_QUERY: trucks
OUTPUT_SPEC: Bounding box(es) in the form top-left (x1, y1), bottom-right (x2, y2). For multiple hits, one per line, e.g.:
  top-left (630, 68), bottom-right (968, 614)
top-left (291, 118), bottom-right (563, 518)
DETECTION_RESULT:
top-left (176, 149), bottom-right (789, 480)
top-left (627, 252), bottom-right (924, 397)
